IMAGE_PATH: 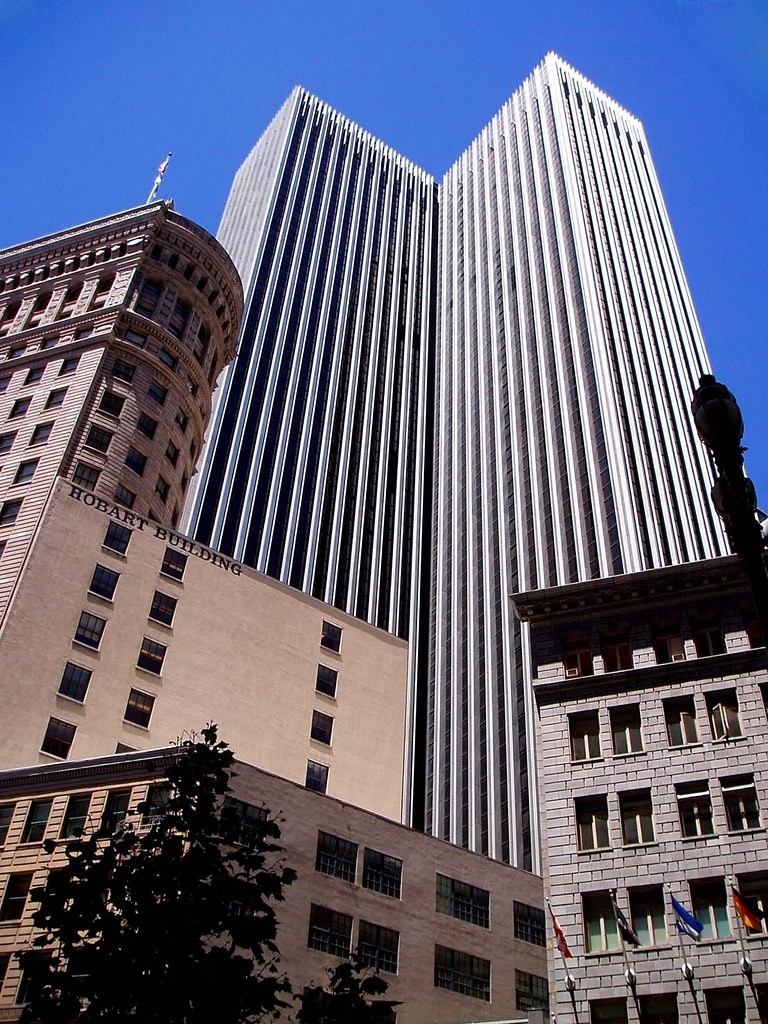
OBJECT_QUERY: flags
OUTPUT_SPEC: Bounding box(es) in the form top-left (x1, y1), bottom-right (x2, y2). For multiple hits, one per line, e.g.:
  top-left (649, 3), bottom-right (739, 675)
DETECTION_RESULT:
top-left (614, 902), bottom-right (642, 946)
top-left (731, 887), bottom-right (764, 931)
top-left (152, 160), bottom-right (168, 199)
top-left (551, 911), bottom-right (573, 959)
top-left (671, 896), bottom-right (704, 941)
